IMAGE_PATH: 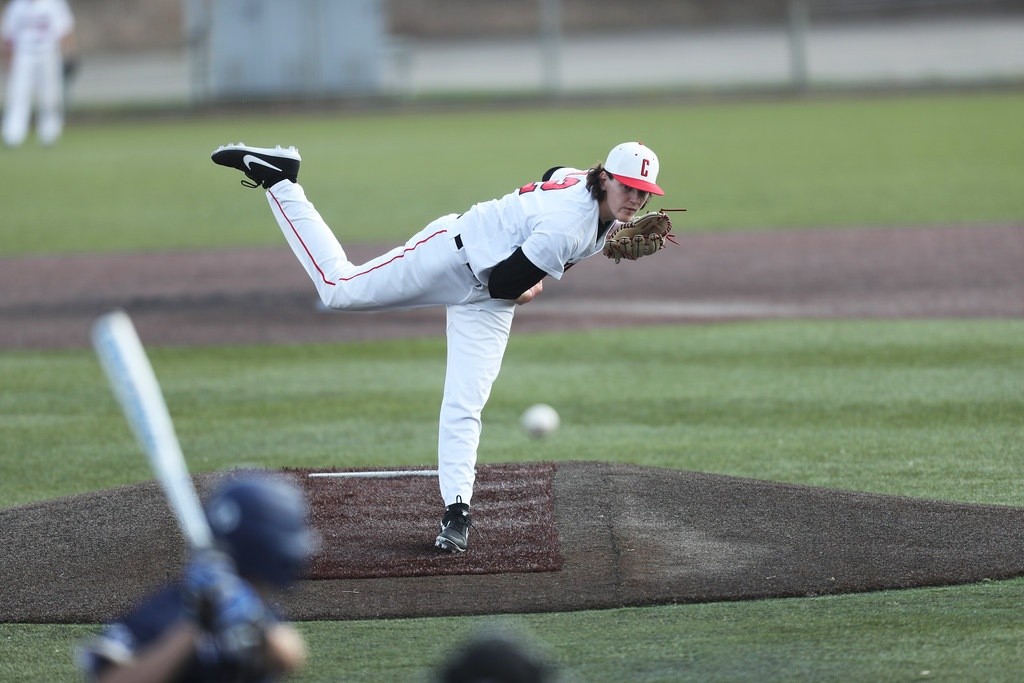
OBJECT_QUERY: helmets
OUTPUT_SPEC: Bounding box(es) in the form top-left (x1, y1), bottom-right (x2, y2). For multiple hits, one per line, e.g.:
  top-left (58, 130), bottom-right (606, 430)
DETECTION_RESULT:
top-left (204, 472), bottom-right (317, 576)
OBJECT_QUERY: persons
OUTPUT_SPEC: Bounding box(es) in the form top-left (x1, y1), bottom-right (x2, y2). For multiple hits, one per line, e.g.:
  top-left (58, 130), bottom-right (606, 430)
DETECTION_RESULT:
top-left (418, 628), bottom-right (575, 683)
top-left (72, 471), bottom-right (313, 683)
top-left (209, 142), bottom-right (674, 553)
top-left (0, 0), bottom-right (77, 150)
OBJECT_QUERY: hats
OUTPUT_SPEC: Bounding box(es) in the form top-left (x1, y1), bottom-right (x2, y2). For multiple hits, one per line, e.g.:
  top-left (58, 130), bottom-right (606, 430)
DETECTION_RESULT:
top-left (603, 141), bottom-right (664, 196)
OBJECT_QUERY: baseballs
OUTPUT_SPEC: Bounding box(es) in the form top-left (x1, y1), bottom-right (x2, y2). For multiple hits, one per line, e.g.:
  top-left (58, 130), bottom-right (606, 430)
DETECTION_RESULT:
top-left (517, 401), bottom-right (563, 442)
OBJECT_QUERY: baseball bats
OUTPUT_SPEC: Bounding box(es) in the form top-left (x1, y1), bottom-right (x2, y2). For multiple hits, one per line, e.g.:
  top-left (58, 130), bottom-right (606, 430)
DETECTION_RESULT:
top-left (83, 304), bottom-right (258, 664)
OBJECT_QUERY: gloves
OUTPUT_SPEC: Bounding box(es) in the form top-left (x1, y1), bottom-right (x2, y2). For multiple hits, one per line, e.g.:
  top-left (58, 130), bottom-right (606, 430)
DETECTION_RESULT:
top-left (174, 554), bottom-right (269, 661)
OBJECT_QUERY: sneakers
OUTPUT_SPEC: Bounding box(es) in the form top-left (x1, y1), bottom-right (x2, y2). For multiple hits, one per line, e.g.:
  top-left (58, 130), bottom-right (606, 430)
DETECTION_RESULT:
top-left (210, 142), bottom-right (301, 190)
top-left (434, 495), bottom-right (479, 554)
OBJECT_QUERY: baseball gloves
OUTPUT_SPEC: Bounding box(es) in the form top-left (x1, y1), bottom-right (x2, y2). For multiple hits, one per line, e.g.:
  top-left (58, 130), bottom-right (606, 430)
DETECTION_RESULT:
top-left (601, 210), bottom-right (673, 264)
